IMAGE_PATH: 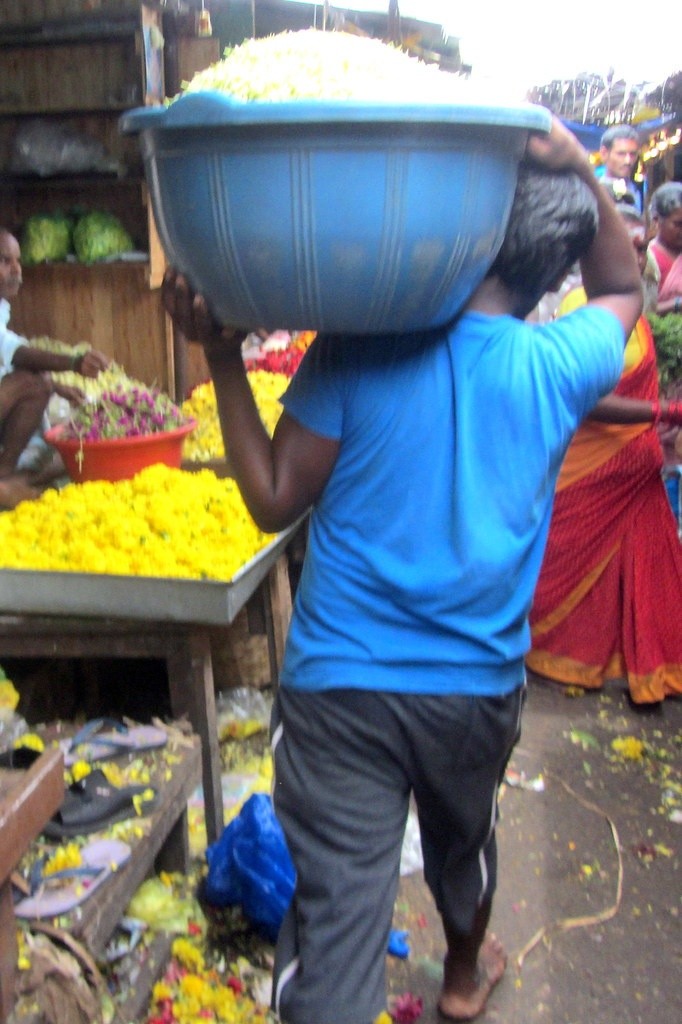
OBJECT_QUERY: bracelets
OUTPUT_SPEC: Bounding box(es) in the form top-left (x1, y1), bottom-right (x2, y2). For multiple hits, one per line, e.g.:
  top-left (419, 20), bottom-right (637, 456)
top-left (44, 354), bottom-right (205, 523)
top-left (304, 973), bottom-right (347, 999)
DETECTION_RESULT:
top-left (72, 354), bottom-right (84, 372)
top-left (674, 295), bottom-right (681, 311)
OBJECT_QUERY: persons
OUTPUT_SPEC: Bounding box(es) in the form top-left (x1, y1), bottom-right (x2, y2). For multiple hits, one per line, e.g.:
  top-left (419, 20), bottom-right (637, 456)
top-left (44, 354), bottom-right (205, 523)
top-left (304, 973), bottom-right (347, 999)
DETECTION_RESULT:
top-left (0, 228), bottom-right (110, 511)
top-left (158, 110), bottom-right (682, 1024)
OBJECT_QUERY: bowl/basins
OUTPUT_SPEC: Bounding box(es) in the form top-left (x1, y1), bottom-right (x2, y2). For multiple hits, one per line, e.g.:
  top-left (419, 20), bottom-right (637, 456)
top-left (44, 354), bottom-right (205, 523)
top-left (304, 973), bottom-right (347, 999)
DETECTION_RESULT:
top-left (117, 88), bottom-right (549, 335)
top-left (44, 410), bottom-right (198, 482)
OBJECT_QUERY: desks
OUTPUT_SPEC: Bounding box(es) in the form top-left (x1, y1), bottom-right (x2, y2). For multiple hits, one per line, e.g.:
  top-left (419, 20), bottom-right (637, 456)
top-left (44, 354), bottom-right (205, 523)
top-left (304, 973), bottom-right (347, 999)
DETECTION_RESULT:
top-left (0, 550), bottom-right (293, 783)
